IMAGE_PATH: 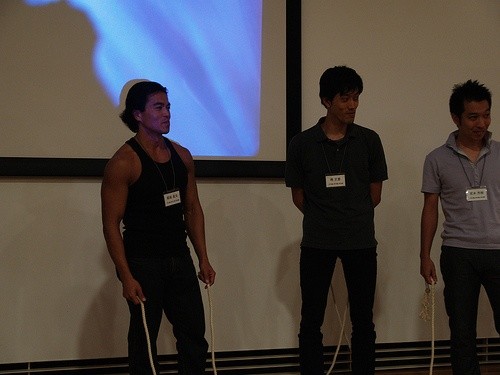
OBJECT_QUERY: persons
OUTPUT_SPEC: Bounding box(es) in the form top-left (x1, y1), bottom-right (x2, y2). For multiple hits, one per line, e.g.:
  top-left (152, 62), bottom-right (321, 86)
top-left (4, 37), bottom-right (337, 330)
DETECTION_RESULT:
top-left (99, 82), bottom-right (218, 375)
top-left (414, 79), bottom-right (500, 374)
top-left (283, 64), bottom-right (391, 375)
